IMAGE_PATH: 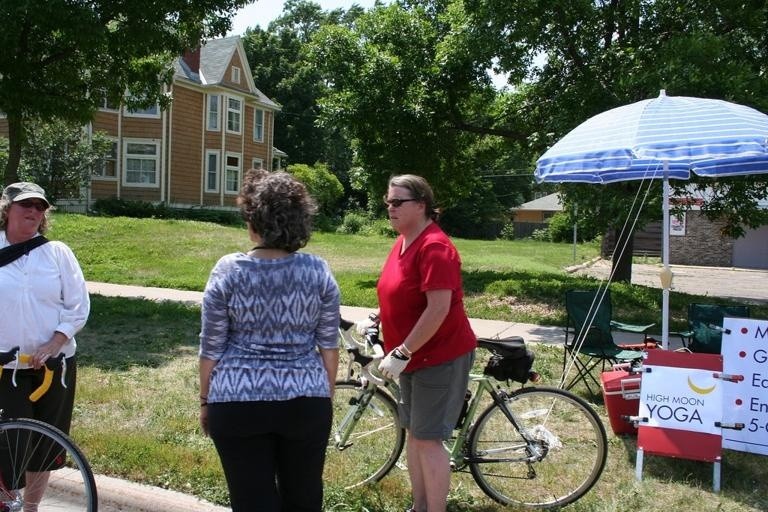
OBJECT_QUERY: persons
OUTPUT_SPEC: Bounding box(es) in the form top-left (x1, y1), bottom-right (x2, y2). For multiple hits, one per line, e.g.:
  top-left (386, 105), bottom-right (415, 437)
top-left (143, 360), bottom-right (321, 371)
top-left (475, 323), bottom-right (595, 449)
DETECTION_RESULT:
top-left (364, 172), bottom-right (481, 511)
top-left (1, 180), bottom-right (92, 512)
top-left (197, 165), bottom-right (343, 511)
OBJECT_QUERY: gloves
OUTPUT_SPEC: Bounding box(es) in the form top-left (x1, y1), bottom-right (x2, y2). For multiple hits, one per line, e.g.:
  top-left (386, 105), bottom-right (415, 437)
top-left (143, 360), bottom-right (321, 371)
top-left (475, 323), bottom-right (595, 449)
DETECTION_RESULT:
top-left (378, 343), bottom-right (413, 380)
top-left (356, 312), bottom-right (381, 336)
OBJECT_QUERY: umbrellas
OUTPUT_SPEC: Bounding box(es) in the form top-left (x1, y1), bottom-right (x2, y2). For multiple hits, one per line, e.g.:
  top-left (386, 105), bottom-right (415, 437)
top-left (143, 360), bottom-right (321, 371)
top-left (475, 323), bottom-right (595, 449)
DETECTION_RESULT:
top-left (531, 86), bottom-right (768, 353)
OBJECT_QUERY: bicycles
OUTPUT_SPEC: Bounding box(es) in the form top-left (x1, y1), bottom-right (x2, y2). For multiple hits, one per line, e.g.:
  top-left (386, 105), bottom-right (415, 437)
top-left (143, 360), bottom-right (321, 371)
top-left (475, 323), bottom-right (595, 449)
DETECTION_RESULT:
top-left (0, 347), bottom-right (98, 512)
top-left (322, 313), bottom-right (607, 508)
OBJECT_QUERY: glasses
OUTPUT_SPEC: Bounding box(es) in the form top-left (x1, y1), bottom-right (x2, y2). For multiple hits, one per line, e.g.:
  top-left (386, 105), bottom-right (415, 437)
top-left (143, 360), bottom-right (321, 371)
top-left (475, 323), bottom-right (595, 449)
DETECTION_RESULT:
top-left (18, 199), bottom-right (46, 211)
top-left (384, 199), bottom-right (416, 208)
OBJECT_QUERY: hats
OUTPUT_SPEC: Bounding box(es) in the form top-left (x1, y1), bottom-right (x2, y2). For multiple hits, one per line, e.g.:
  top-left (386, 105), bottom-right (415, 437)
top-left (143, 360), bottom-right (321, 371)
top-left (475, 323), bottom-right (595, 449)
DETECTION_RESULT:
top-left (1, 182), bottom-right (50, 208)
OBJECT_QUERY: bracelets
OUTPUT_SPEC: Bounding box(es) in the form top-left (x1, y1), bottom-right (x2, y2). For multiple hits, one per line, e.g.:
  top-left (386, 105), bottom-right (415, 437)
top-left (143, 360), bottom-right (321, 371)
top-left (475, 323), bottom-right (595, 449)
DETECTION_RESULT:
top-left (401, 343), bottom-right (413, 354)
top-left (199, 396), bottom-right (208, 407)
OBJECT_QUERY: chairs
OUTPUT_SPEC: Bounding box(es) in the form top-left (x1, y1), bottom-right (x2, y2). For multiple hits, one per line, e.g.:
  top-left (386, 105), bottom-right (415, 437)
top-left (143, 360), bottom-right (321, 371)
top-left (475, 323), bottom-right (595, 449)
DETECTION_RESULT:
top-left (560, 286), bottom-right (644, 404)
top-left (669, 303), bottom-right (750, 354)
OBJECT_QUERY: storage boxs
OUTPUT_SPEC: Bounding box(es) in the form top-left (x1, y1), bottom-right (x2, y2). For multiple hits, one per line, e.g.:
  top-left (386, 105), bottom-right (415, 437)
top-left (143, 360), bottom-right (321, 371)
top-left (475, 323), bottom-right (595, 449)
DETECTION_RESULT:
top-left (600, 363), bottom-right (642, 434)
